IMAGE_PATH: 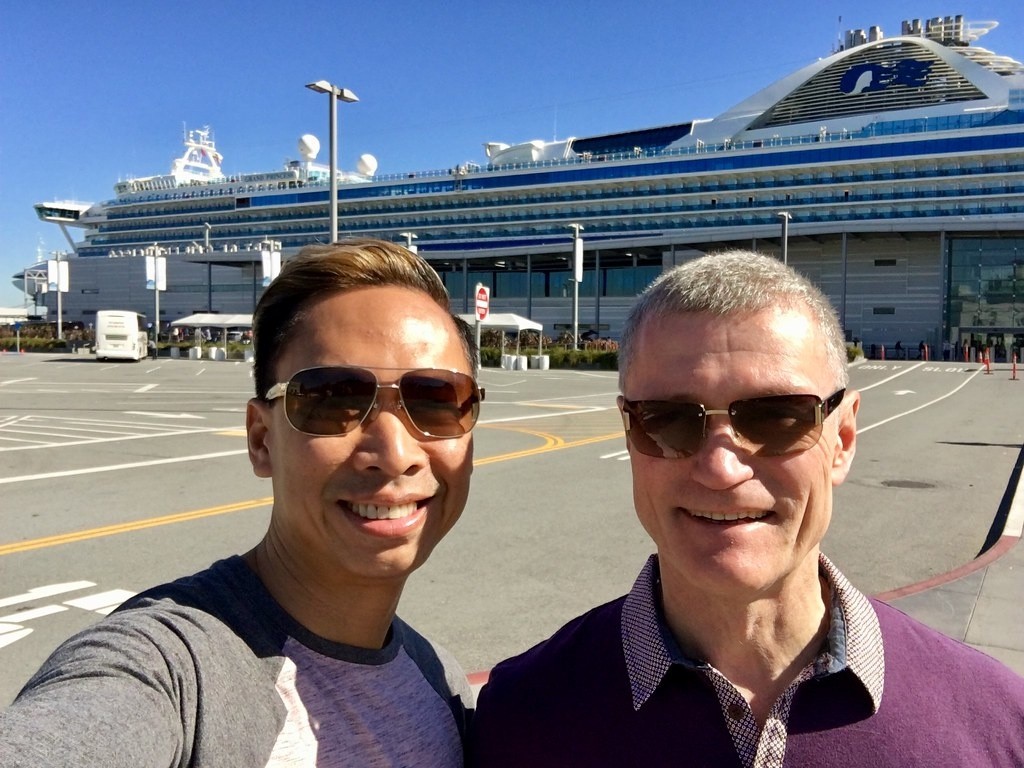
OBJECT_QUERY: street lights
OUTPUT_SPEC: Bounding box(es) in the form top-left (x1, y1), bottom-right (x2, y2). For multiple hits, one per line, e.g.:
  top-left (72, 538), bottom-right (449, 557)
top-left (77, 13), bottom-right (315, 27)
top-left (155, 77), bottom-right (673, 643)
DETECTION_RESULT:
top-left (569, 222), bottom-right (585, 349)
top-left (399, 231), bottom-right (418, 248)
top-left (149, 245), bottom-right (160, 347)
top-left (261, 239), bottom-right (279, 286)
top-left (49, 251), bottom-right (68, 338)
top-left (778, 212), bottom-right (792, 266)
top-left (305, 79), bottom-right (360, 242)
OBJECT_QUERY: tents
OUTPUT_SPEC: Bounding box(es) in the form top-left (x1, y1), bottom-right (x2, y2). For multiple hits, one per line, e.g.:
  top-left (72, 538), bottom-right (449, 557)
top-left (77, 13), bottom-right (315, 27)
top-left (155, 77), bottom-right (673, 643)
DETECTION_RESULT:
top-left (457, 313), bottom-right (543, 356)
top-left (171, 313), bottom-right (254, 360)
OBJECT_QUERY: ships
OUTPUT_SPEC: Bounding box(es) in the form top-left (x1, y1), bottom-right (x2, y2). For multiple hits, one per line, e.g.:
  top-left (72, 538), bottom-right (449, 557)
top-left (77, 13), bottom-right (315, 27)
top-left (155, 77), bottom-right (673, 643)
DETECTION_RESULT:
top-left (11, 17), bottom-right (1023, 360)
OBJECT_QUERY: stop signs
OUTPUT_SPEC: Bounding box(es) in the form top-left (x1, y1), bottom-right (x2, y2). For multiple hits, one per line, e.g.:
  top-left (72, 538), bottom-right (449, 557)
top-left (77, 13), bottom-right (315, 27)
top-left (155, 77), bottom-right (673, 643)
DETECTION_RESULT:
top-left (474, 284), bottom-right (491, 322)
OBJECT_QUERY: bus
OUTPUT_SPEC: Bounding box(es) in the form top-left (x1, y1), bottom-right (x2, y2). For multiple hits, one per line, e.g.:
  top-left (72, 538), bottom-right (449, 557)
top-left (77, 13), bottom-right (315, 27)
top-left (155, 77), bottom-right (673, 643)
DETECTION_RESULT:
top-left (94, 309), bottom-right (148, 363)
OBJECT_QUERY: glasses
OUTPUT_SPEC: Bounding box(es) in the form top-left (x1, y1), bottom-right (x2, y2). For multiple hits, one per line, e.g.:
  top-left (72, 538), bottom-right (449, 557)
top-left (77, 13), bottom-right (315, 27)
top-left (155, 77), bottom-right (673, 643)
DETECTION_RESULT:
top-left (264, 367), bottom-right (486, 439)
top-left (622, 387), bottom-right (845, 461)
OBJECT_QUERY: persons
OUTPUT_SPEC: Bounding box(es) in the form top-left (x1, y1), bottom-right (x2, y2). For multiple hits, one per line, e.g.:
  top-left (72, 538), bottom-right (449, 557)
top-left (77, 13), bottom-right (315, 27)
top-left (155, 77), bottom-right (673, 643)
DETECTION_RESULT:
top-left (468, 251), bottom-right (1024, 768)
top-left (852, 338), bottom-right (1024, 362)
top-left (0, 235), bottom-right (485, 768)
top-left (147, 322), bottom-right (253, 360)
